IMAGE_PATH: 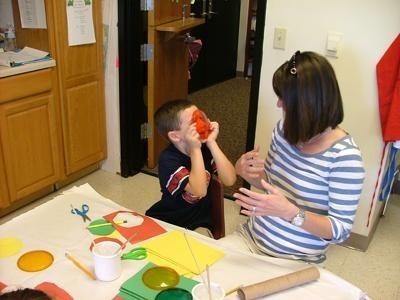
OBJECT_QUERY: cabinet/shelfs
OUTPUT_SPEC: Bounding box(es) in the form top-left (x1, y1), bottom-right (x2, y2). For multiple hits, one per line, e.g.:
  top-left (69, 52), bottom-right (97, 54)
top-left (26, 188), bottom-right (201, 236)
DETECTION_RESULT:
top-left (5, 23), bottom-right (16, 51)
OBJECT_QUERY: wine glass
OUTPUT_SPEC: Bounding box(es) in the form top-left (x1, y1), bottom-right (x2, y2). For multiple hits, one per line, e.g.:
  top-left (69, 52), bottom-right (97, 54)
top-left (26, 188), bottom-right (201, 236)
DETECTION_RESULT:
top-left (253, 207), bottom-right (256, 213)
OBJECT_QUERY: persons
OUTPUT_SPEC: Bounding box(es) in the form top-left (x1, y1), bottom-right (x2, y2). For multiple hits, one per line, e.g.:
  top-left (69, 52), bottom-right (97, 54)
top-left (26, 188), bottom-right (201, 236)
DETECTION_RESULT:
top-left (216, 49), bottom-right (366, 267)
top-left (145, 98), bottom-right (237, 238)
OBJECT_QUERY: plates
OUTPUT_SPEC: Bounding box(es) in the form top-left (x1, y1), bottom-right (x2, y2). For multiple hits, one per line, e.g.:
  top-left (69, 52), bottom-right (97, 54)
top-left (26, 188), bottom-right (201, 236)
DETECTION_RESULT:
top-left (0, 68), bottom-right (60, 217)
top-left (11, 0), bottom-right (108, 191)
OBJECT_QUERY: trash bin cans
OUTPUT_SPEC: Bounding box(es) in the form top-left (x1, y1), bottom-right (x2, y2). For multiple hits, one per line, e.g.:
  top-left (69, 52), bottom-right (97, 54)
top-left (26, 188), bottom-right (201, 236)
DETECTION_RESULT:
top-left (290, 209), bottom-right (307, 230)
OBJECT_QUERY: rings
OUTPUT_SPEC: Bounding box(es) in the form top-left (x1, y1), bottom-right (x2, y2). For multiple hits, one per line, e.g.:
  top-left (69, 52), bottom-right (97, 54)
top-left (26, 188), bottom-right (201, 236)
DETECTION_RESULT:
top-left (86, 220), bottom-right (127, 229)
top-left (65, 252), bottom-right (96, 281)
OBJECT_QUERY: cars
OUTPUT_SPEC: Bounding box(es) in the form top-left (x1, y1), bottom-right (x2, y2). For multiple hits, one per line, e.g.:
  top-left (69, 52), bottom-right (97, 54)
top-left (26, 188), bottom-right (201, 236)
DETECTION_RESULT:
top-left (91, 241), bottom-right (122, 282)
top-left (192, 283), bottom-right (226, 300)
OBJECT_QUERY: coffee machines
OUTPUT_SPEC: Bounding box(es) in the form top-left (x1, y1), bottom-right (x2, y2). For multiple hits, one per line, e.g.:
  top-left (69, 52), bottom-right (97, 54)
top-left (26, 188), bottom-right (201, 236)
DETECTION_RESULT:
top-left (71, 204), bottom-right (91, 223)
top-left (121, 247), bottom-right (148, 260)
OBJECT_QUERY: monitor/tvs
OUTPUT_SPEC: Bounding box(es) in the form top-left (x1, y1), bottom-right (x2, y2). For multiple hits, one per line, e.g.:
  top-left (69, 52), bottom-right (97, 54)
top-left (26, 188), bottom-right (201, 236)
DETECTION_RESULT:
top-left (0, 46), bottom-right (49, 68)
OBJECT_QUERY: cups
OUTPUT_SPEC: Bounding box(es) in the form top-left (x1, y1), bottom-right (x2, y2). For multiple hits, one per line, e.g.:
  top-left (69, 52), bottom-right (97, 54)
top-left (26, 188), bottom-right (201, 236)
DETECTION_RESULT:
top-left (286, 50), bottom-right (300, 84)
